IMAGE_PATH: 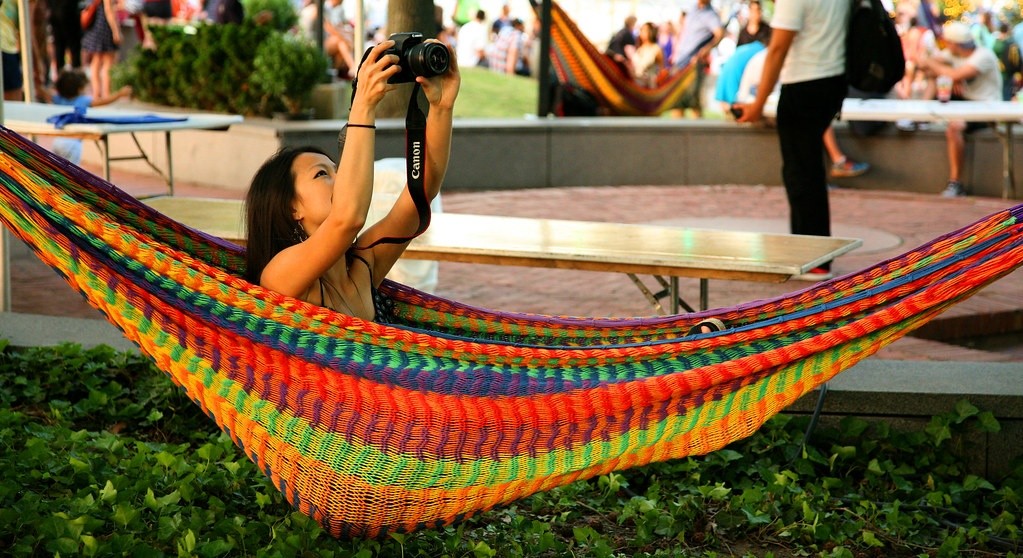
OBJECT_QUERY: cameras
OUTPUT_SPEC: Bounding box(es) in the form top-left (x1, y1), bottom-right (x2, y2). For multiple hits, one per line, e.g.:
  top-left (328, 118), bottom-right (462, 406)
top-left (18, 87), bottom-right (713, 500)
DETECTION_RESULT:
top-left (375, 32), bottom-right (450, 84)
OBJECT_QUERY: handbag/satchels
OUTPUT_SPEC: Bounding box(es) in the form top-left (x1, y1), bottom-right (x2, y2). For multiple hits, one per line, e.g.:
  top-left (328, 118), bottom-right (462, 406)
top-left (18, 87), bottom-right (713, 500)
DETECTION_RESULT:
top-left (80, 0), bottom-right (101, 31)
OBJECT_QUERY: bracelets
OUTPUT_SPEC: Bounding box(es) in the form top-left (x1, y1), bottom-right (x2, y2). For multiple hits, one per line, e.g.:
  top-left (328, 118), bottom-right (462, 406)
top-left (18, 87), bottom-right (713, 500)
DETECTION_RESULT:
top-left (345, 124), bottom-right (376, 130)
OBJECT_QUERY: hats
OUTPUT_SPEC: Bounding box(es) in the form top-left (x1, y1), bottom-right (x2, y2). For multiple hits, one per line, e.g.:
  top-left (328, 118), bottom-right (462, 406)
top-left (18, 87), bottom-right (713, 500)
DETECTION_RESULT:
top-left (939, 20), bottom-right (975, 50)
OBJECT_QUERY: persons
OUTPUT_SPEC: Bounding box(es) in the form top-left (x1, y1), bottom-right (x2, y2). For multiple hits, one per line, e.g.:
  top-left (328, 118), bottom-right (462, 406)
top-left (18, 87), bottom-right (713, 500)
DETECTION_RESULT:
top-left (39, 70), bottom-right (134, 170)
top-left (909, 18), bottom-right (1007, 200)
top-left (728, 1), bottom-right (854, 287)
top-left (736, 47), bottom-right (872, 178)
top-left (47, 1), bottom-right (1023, 99)
top-left (244, 38), bottom-right (461, 329)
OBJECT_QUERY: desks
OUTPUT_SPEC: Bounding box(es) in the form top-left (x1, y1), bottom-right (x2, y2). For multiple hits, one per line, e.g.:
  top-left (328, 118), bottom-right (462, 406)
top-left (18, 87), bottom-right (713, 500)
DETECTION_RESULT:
top-left (3, 106), bottom-right (243, 202)
top-left (826, 92), bottom-right (1022, 202)
top-left (129, 195), bottom-right (868, 320)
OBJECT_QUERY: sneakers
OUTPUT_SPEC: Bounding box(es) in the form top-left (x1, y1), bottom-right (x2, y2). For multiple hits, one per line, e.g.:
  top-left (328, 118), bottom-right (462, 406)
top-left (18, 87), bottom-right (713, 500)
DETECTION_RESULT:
top-left (830, 155), bottom-right (871, 177)
top-left (940, 180), bottom-right (967, 197)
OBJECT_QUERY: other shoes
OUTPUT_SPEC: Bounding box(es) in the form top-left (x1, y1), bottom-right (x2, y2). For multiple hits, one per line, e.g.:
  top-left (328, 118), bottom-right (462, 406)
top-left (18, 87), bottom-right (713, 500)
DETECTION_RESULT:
top-left (789, 267), bottom-right (833, 281)
top-left (682, 317), bottom-right (726, 338)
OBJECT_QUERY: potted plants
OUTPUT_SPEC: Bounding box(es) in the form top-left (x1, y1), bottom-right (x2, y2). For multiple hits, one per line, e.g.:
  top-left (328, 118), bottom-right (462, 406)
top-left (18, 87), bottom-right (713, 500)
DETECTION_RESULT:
top-left (253, 34), bottom-right (334, 122)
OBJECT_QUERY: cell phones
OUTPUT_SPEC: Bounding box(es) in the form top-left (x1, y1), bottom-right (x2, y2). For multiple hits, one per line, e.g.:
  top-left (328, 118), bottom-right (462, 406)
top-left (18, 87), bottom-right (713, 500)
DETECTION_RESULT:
top-left (731, 109), bottom-right (741, 120)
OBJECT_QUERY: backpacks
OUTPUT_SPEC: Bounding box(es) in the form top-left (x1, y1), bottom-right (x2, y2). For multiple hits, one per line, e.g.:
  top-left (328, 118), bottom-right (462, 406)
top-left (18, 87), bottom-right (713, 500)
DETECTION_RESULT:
top-left (847, 0), bottom-right (905, 94)
